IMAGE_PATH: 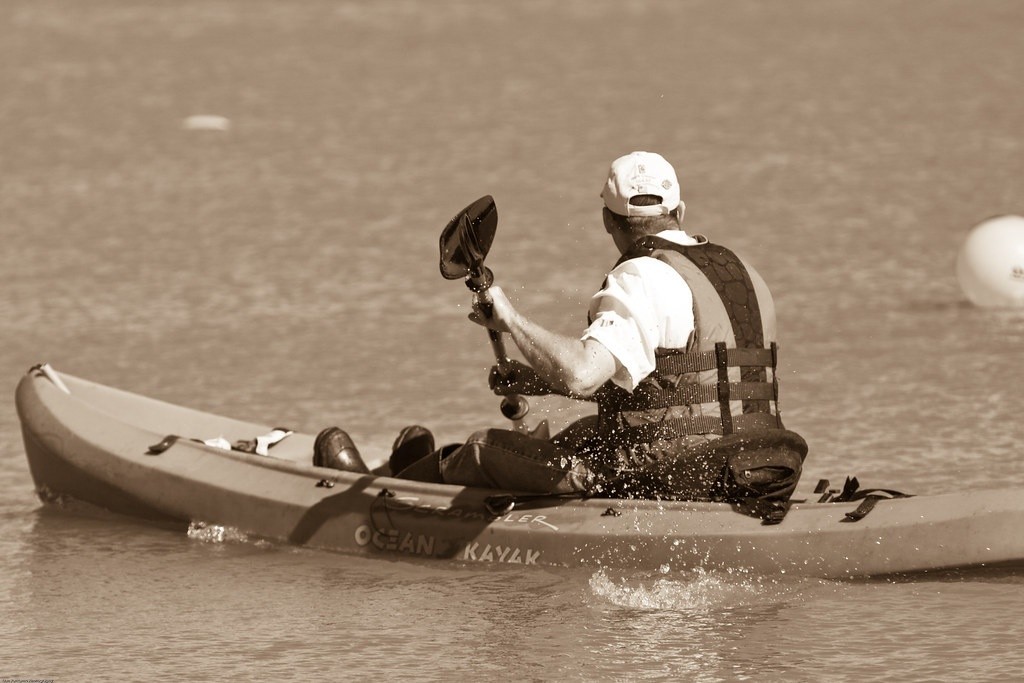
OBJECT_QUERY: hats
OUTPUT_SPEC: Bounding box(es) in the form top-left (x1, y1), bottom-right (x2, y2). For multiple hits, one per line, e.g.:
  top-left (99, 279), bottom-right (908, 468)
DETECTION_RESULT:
top-left (599, 151), bottom-right (680, 216)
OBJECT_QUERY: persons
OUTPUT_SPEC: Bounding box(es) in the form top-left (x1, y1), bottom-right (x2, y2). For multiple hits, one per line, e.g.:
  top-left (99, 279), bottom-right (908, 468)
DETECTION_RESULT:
top-left (313, 150), bottom-right (785, 495)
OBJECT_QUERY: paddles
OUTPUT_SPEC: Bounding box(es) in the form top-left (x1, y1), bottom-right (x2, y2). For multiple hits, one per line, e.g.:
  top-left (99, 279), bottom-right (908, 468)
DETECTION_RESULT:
top-left (436, 192), bottom-right (534, 436)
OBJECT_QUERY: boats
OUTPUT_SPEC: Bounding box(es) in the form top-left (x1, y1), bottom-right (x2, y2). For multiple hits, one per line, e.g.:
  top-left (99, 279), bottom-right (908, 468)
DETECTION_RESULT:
top-left (16, 360), bottom-right (1024, 585)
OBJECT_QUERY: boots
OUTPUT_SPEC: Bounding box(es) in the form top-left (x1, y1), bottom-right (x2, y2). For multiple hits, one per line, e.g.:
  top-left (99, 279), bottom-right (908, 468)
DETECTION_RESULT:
top-left (314, 425), bottom-right (464, 485)
top-left (388, 424), bottom-right (433, 475)
